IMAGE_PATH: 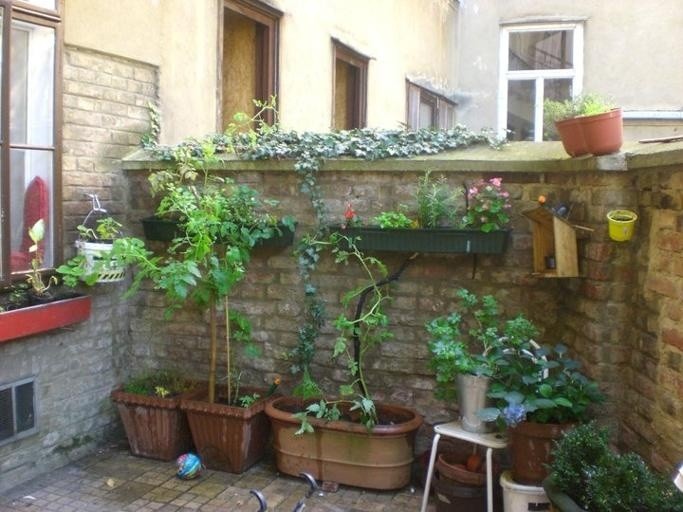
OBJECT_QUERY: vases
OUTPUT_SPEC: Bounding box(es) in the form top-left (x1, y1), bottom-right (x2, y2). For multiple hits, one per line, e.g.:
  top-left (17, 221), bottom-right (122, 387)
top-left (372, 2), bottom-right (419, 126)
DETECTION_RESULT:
top-left (605, 208), bottom-right (639, 243)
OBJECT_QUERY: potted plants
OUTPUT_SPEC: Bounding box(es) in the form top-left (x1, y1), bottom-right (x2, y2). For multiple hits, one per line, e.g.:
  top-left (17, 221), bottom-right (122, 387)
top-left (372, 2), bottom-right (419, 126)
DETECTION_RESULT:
top-left (275, 158), bottom-right (542, 509)
top-left (76, 204), bottom-right (128, 285)
top-left (487, 342), bottom-right (682, 510)
top-left (110, 157), bottom-right (275, 475)
top-left (572, 92), bottom-right (623, 157)
top-left (541, 92), bottom-right (593, 159)
